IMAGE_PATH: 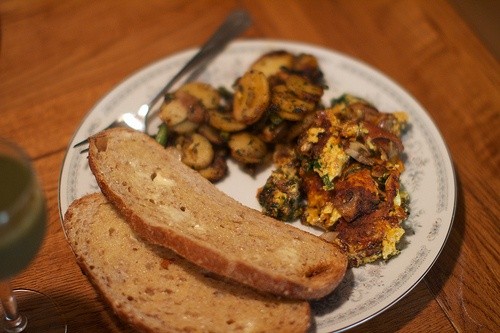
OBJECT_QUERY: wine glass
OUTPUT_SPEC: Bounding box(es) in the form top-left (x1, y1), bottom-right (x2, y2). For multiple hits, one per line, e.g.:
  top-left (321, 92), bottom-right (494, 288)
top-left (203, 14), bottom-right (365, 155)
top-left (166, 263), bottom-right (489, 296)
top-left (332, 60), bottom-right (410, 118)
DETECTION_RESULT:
top-left (1, 138), bottom-right (69, 333)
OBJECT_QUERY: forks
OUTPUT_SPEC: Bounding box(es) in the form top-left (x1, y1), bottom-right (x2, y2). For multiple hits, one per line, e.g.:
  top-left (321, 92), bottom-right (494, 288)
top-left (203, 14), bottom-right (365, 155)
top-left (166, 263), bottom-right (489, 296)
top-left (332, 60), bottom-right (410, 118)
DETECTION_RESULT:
top-left (74, 9), bottom-right (254, 159)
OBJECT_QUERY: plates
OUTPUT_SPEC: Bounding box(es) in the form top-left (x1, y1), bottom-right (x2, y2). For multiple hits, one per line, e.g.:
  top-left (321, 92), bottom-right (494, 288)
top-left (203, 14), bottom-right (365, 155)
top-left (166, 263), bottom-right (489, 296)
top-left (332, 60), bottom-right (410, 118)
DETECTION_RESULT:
top-left (55, 38), bottom-right (457, 333)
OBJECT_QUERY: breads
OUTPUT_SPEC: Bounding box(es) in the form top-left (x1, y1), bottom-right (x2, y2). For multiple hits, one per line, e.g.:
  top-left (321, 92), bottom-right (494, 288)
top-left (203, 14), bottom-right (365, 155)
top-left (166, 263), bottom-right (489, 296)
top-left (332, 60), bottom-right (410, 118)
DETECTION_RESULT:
top-left (88, 126), bottom-right (348, 299)
top-left (63, 190), bottom-right (314, 333)
